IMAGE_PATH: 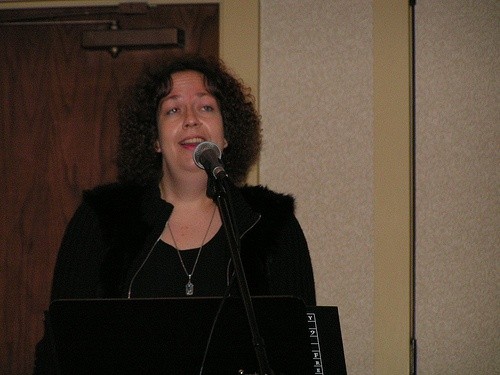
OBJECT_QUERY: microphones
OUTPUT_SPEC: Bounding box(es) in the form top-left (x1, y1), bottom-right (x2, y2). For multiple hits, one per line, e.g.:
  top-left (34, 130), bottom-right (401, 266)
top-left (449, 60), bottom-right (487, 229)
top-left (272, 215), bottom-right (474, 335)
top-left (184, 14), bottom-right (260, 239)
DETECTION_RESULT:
top-left (192, 140), bottom-right (231, 194)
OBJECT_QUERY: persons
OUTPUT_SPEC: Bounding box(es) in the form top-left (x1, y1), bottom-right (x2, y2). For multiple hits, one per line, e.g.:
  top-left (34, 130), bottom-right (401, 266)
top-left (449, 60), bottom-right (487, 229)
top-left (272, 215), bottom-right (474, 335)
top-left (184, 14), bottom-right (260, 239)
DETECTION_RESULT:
top-left (167, 204), bottom-right (218, 296)
top-left (33, 53), bottom-right (317, 375)
